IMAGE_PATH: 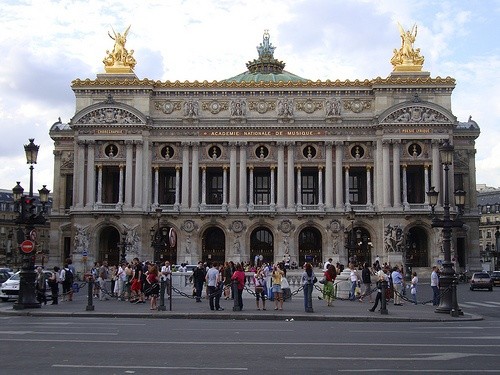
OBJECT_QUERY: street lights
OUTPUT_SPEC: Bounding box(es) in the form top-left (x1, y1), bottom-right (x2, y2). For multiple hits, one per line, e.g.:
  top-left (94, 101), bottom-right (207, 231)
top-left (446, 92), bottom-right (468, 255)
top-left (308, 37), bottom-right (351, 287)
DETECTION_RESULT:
top-left (12, 139), bottom-right (50, 309)
top-left (150, 203), bottom-right (167, 268)
top-left (344, 205), bottom-right (361, 294)
top-left (423, 137), bottom-right (467, 312)
top-left (117, 230), bottom-right (132, 266)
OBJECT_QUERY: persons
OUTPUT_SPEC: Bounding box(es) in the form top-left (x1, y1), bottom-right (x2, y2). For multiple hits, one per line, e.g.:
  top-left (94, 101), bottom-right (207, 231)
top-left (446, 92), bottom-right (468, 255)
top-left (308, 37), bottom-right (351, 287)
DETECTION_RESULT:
top-left (172, 263), bottom-right (187, 272)
top-left (91, 257), bottom-right (172, 310)
top-left (300, 262), bottom-right (317, 313)
top-left (103, 31), bottom-right (137, 67)
top-left (189, 261), bottom-right (245, 311)
top-left (323, 258), bottom-right (343, 306)
top-left (36, 258), bottom-right (75, 305)
top-left (283, 255), bottom-right (298, 270)
top-left (430, 266), bottom-right (443, 306)
top-left (390, 26), bottom-right (424, 65)
top-left (254, 254), bottom-right (263, 267)
top-left (244, 261), bottom-right (286, 311)
top-left (409, 272), bottom-right (418, 305)
top-left (348, 256), bottom-right (406, 312)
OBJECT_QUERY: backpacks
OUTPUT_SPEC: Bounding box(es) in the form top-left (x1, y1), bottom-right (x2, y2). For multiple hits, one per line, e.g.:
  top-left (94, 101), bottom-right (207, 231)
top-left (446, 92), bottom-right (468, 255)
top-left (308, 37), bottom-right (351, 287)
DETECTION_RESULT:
top-left (62, 268), bottom-right (73, 284)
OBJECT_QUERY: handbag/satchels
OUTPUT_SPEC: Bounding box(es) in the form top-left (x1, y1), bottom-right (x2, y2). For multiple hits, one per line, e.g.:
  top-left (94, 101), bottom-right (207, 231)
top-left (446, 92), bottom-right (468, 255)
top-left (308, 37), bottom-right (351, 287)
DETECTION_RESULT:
top-left (312, 277), bottom-right (317, 283)
top-left (319, 276), bottom-right (328, 284)
top-left (280, 274), bottom-right (290, 289)
top-left (47, 278), bottom-right (56, 286)
top-left (255, 287), bottom-right (264, 292)
top-left (411, 287), bottom-right (416, 295)
top-left (355, 287), bottom-right (361, 297)
top-left (36, 291), bottom-right (45, 303)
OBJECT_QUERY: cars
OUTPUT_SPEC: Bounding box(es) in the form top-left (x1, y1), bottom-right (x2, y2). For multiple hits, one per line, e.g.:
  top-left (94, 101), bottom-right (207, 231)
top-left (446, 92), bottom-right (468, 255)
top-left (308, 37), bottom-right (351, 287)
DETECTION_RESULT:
top-left (0, 268), bottom-right (13, 286)
top-left (491, 271), bottom-right (500, 287)
top-left (469, 271), bottom-right (492, 291)
top-left (0, 269), bottom-right (58, 302)
top-left (162, 264), bottom-right (209, 274)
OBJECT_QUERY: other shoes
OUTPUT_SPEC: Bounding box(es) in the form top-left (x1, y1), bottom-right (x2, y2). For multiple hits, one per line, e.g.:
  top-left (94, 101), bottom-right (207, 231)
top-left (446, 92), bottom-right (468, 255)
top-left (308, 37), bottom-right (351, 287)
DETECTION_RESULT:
top-left (369, 309), bottom-right (374, 311)
top-left (216, 308), bottom-right (224, 310)
top-left (274, 307), bottom-right (278, 310)
top-left (196, 300), bottom-right (201, 302)
top-left (93, 293), bottom-right (158, 310)
top-left (395, 304), bottom-right (403, 306)
top-left (359, 299), bottom-right (366, 303)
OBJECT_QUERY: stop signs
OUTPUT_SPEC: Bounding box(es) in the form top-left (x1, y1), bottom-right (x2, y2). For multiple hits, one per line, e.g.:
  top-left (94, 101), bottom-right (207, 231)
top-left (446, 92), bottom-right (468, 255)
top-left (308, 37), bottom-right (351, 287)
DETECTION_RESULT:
top-left (21, 240), bottom-right (34, 254)
